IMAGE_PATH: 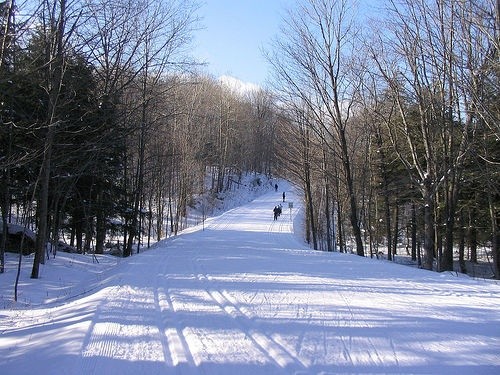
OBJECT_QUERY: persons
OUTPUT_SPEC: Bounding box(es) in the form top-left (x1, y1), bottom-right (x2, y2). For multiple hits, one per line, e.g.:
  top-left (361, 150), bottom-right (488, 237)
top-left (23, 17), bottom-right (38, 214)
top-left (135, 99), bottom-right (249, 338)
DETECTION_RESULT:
top-left (282, 192), bottom-right (286, 202)
top-left (274, 183), bottom-right (278, 192)
top-left (273, 206), bottom-right (278, 220)
top-left (280, 203), bottom-right (283, 212)
top-left (277, 205), bottom-right (280, 216)
